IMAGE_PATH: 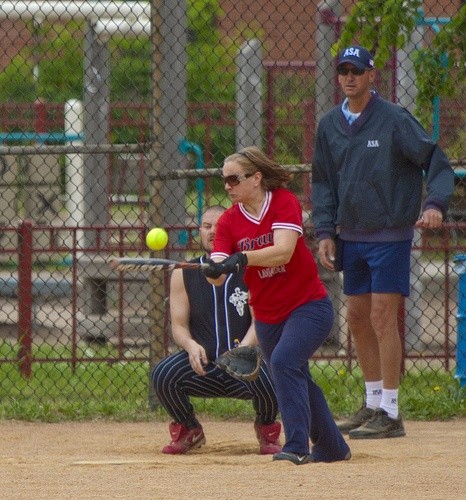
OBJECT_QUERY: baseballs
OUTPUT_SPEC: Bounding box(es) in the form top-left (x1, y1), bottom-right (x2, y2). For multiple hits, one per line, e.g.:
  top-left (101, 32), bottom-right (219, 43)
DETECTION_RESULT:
top-left (146, 228), bottom-right (168, 251)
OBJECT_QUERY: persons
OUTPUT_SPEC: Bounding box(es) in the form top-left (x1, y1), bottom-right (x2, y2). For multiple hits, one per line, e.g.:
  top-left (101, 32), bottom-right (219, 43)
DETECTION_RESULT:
top-left (202, 146), bottom-right (351, 465)
top-left (153, 205), bottom-right (283, 455)
top-left (311, 46), bottom-right (454, 440)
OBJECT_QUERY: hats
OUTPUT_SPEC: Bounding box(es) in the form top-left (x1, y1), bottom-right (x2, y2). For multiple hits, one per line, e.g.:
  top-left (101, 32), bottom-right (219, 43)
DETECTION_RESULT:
top-left (337, 45), bottom-right (375, 70)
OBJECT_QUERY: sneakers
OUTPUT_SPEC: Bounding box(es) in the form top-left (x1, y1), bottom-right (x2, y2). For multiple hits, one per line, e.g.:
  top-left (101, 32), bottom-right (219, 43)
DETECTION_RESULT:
top-left (308, 447), bottom-right (351, 463)
top-left (335, 401), bottom-right (376, 432)
top-left (348, 407), bottom-right (405, 439)
top-left (253, 420), bottom-right (283, 454)
top-left (273, 450), bottom-right (309, 465)
top-left (162, 422), bottom-right (207, 454)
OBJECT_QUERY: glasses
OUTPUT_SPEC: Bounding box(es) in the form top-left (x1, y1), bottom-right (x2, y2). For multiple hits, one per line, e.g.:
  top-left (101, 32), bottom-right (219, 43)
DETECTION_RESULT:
top-left (337, 67), bottom-right (371, 76)
top-left (221, 170), bottom-right (258, 187)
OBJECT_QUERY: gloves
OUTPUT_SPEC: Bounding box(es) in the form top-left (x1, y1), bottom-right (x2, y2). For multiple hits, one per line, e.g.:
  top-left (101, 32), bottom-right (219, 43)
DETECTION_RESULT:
top-left (220, 251), bottom-right (248, 273)
top-left (202, 258), bottom-right (223, 279)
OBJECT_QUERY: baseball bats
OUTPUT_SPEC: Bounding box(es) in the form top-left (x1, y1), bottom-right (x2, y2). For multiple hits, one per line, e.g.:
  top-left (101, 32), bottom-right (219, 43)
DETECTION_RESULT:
top-left (109, 258), bottom-right (240, 274)
